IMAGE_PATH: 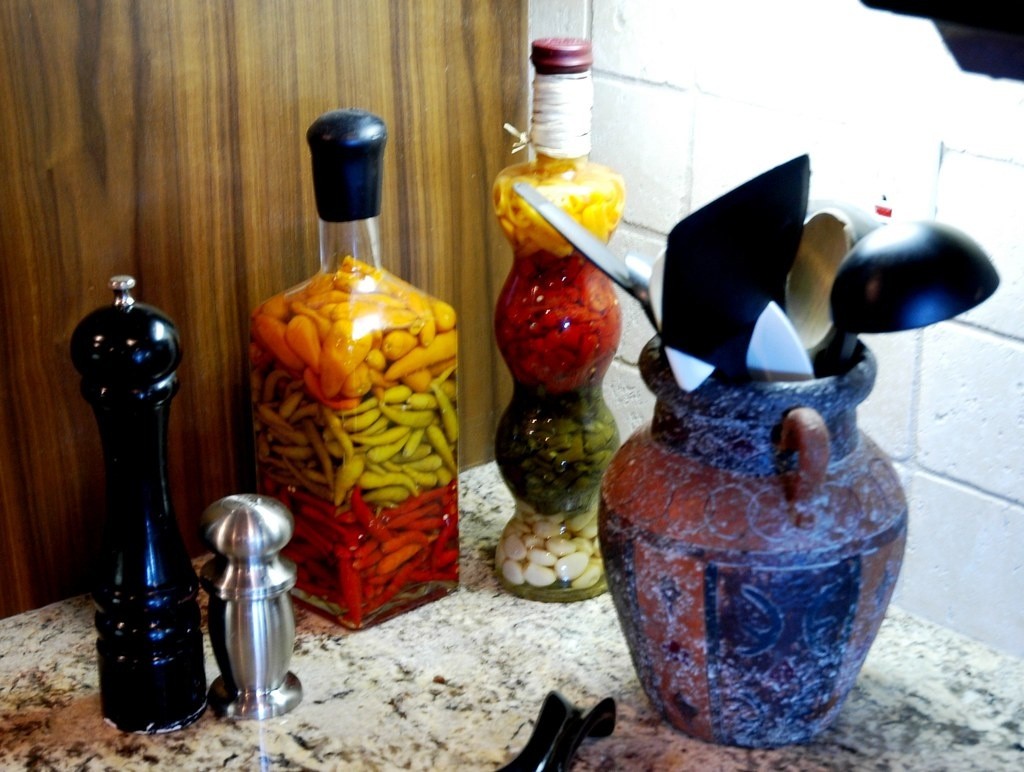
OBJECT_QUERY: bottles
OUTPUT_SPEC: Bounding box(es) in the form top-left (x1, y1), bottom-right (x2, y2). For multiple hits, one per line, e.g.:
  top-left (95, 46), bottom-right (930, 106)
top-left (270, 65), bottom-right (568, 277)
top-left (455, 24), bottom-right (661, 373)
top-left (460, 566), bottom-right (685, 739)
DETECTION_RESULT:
top-left (492, 34), bottom-right (623, 604)
top-left (239, 112), bottom-right (462, 628)
top-left (198, 494), bottom-right (300, 722)
top-left (598, 341), bottom-right (913, 749)
top-left (68, 274), bottom-right (205, 735)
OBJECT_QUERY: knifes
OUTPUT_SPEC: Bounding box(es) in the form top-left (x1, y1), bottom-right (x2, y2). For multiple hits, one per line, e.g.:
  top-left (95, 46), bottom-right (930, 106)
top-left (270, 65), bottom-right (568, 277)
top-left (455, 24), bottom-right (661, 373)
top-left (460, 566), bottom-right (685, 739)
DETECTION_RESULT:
top-left (509, 183), bottom-right (659, 341)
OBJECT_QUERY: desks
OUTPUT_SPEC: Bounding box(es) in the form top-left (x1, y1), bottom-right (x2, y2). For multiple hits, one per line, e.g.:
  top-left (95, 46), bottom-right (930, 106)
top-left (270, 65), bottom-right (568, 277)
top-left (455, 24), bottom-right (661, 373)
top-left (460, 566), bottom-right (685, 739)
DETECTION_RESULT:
top-left (0, 461), bottom-right (1024, 772)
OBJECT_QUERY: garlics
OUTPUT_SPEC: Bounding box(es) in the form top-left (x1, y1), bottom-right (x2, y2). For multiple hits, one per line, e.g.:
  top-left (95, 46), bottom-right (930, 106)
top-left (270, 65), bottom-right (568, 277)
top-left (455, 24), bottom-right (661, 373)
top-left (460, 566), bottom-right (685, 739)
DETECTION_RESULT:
top-left (494, 503), bottom-right (604, 590)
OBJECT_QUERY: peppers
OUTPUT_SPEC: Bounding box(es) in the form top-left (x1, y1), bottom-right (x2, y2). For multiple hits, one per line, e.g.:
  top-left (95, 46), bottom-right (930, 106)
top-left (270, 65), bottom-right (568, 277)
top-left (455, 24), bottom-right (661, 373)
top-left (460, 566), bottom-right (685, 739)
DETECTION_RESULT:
top-left (246, 258), bottom-right (459, 630)
